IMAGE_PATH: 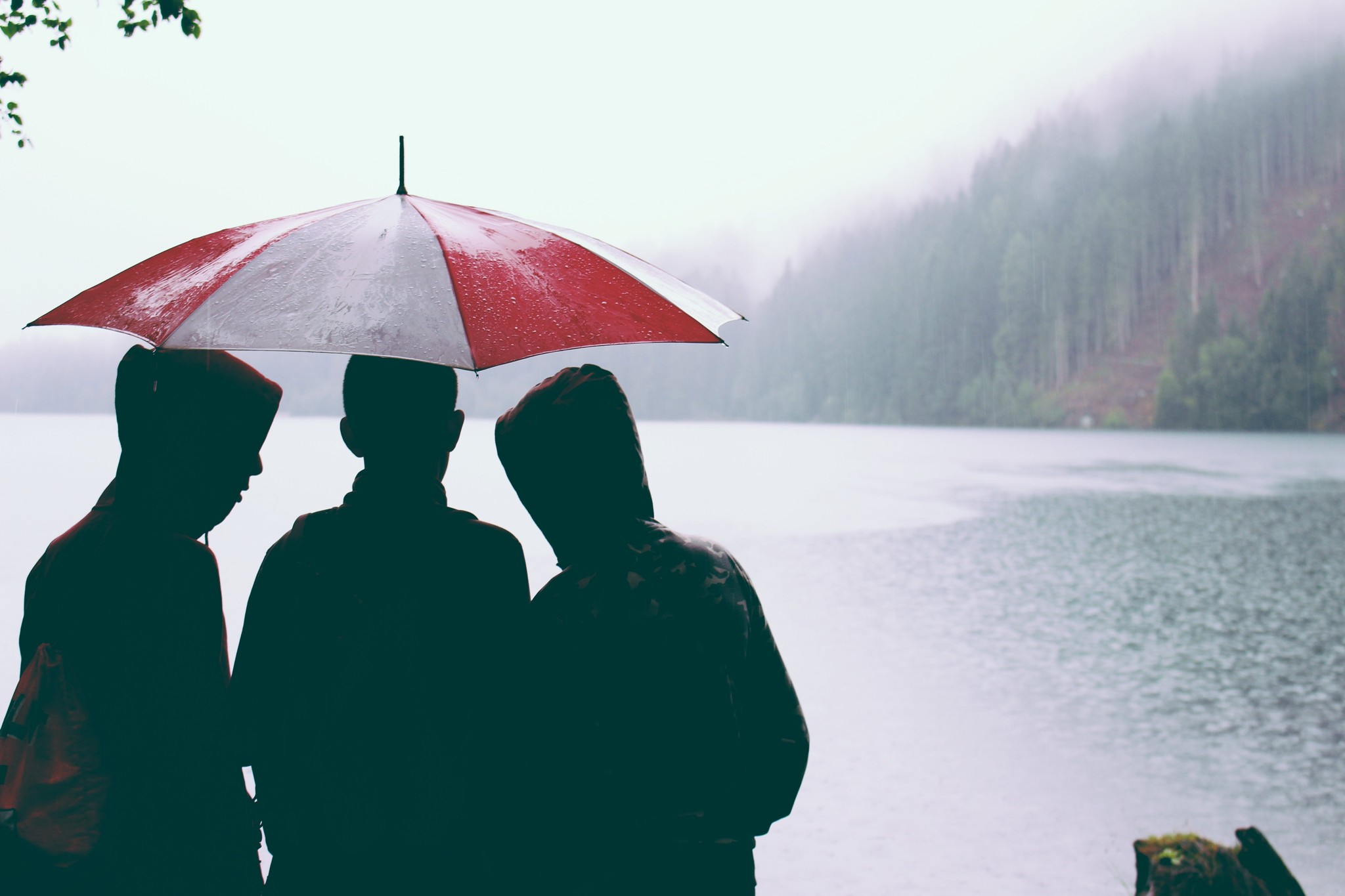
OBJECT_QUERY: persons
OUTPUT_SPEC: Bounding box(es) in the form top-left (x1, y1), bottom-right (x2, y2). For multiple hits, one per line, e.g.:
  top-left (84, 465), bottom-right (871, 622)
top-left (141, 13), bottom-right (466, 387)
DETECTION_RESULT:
top-left (1132, 834), bottom-right (1271, 895)
top-left (1, 341), bottom-right (287, 896)
top-left (493, 363), bottom-right (809, 896)
top-left (228, 351), bottom-right (539, 896)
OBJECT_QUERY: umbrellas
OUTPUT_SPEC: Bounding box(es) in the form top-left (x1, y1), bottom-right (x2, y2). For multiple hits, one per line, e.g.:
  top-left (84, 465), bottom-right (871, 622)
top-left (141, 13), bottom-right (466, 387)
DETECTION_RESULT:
top-left (18, 133), bottom-right (751, 381)
